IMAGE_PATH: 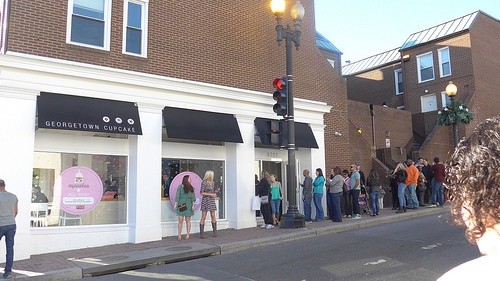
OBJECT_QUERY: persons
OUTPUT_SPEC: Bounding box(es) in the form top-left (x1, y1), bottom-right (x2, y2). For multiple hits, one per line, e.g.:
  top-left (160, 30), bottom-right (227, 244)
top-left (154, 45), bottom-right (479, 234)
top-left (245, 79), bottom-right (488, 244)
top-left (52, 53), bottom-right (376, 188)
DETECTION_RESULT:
top-left (200, 170), bottom-right (220, 238)
top-left (173, 175), bottom-right (196, 241)
top-left (435, 115), bottom-right (500, 281)
top-left (366, 167), bottom-right (381, 216)
top-left (389, 157), bottom-right (446, 213)
top-left (33, 187), bottom-right (48, 203)
top-left (103, 179), bottom-right (118, 198)
top-left (0, 179), bottom-right (18, 279)
top-left (313, 168), bottom-right (325, 221)
top-left (299, 170), bottom-right (313, 223)
top-left (270, 174), bottom-right (284, 225)
top-left (259, 170), bottom-right (274, 228)
top-left (163, 174), bottom-right (171, 197)
top-left (325, 164), bottom-right (366, 222)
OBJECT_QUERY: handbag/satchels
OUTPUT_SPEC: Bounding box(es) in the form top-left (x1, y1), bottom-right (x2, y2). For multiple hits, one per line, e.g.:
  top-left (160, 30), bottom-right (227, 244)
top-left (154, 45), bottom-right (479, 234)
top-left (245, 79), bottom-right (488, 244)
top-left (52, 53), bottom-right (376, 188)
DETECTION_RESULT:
top-left (178, 204), bottom-right (188, 212)
top-left (260, 195), bottom-right (269, 204)
top-left (252, 196), bottom-right (261, 210)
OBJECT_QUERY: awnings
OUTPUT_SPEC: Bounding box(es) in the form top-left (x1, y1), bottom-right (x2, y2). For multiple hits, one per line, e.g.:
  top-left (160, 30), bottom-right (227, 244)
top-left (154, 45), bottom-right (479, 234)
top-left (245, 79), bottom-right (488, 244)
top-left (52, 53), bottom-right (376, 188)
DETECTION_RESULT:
top-left (162, 105), bottom-right (243, 142)
top-left (252, 117), bottom-right (320, 149)
top-left (34, 92), bottom-right (143, 136)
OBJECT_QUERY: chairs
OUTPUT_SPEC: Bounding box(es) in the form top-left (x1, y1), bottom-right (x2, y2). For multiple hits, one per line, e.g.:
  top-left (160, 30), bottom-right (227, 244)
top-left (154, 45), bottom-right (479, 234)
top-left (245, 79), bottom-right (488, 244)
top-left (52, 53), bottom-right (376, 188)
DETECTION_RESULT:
top-left (30, 203), bottom-right (82, 227)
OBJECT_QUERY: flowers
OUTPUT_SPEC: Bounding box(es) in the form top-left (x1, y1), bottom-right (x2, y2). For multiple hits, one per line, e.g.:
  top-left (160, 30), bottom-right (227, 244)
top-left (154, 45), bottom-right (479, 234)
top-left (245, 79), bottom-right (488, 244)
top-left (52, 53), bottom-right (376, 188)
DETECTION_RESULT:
top-left (437, 100), bottom-right (474, 126)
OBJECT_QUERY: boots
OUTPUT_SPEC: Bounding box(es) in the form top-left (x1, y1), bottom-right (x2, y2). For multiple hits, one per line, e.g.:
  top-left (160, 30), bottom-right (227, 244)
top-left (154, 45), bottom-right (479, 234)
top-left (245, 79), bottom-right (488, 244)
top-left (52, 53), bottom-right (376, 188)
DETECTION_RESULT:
top-left (199, 224), bottom-right (206, 239)
top-left (211, 221), bottom-right (217, 237)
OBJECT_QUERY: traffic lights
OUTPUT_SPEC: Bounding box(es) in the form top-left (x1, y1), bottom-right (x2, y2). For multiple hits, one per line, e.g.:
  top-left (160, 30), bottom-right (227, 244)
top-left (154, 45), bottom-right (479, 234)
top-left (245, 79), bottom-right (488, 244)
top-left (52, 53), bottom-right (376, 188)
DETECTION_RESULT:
top-left (272, 77), bottom-right (288, 116)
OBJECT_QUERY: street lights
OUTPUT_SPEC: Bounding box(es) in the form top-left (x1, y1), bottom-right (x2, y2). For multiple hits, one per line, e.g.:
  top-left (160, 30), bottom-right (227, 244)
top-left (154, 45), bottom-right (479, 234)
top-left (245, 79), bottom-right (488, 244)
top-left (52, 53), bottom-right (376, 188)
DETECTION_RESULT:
top-left (270, 0), bottom-right (306, 229)
top-left (445, 80), bottom-right (458, 150)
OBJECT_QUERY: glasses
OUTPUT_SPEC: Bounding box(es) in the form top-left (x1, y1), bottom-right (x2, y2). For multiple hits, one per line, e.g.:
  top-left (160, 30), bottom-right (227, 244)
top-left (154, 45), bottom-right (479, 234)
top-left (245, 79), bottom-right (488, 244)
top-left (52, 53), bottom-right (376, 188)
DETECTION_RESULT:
top-left (343, 174), bottom-right (347, 175)
top-left (351, 166), bottom-right (355, 168)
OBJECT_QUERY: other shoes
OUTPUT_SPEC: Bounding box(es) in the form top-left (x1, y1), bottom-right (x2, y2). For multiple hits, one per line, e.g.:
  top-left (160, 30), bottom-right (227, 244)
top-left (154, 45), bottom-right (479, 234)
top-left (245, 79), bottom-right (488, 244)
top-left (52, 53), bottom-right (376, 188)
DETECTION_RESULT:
top-left (419, 203), bottom-right (426, 207)
top-left (395, 209), bottom-right (403, 213)
top-left (353, 214), bottom-right (362, 219)
top-left (348, 215), bottom-right (351, 218)
top-left (343, 214), bottom-right (347, 218)
top-left (404, 208), bottom-right (407, 212)
top-left (177, 235), bottom-right (182, 240)
top-left (3, 273), bottom-right (12, 278)
top-left (377, 212), bottom-right (379, 215)
top-left (392, 207), bottom-right (399, 210)
top-left (276, 220), bottom-right (279, 225)
top-left (373, 213), bottom-right (376, 215)
top-left (260, 223), bottom-right (267, 227)
top-left (305, 220), bottom-right (312, 224)
top-left (428, 204), bottom-right (437, 208)
top-left (313, 218), bottom-right (323, 222)
top-left (185, 235), bottom-right (190, 240)
top-left (266, 224), bottom-right (275, 229)
